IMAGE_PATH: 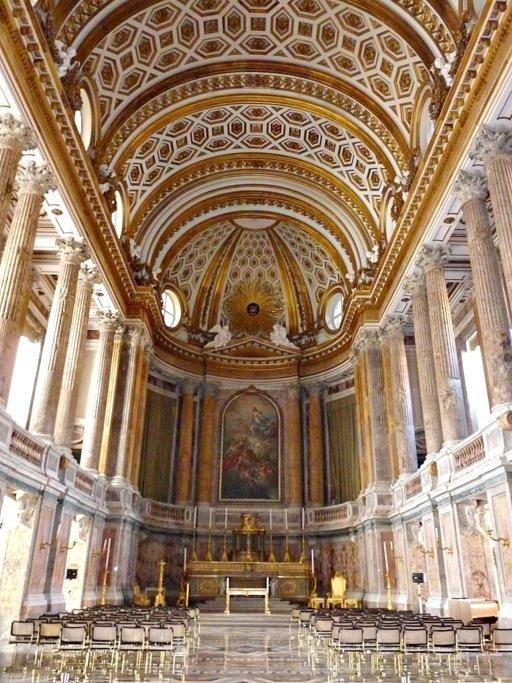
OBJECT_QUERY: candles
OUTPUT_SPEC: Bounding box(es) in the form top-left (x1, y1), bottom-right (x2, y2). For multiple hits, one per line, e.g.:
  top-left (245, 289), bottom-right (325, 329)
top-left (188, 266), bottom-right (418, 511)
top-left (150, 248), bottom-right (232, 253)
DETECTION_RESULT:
top-left (267, 508), bottom-right (273, 530)
top-left (300, 506), bottom-right (306, 531)
top-left (284, 508), bottom-right (288, 530)
top-left (193, 503), bottom-right (197, 531)
top-left (207, 505), bottom-right (212, 532)
top-left (223, 506), bottom-right (229, 531)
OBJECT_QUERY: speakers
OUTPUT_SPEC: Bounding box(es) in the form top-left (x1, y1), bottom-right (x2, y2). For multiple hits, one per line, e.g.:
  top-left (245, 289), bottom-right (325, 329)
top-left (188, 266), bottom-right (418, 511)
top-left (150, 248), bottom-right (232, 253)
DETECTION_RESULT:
top-left (412, 573), bottom-right (423, 583)
top-left (66, 569), bottom-right (77, 578)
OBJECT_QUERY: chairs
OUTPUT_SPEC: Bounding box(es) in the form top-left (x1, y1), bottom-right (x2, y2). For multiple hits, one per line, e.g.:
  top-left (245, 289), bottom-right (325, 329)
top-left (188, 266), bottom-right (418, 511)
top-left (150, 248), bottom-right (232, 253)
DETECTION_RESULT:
top-left (7, 604), bottom-right (202, 674)
top-left (288, 607), bottom-right (499, 679)
